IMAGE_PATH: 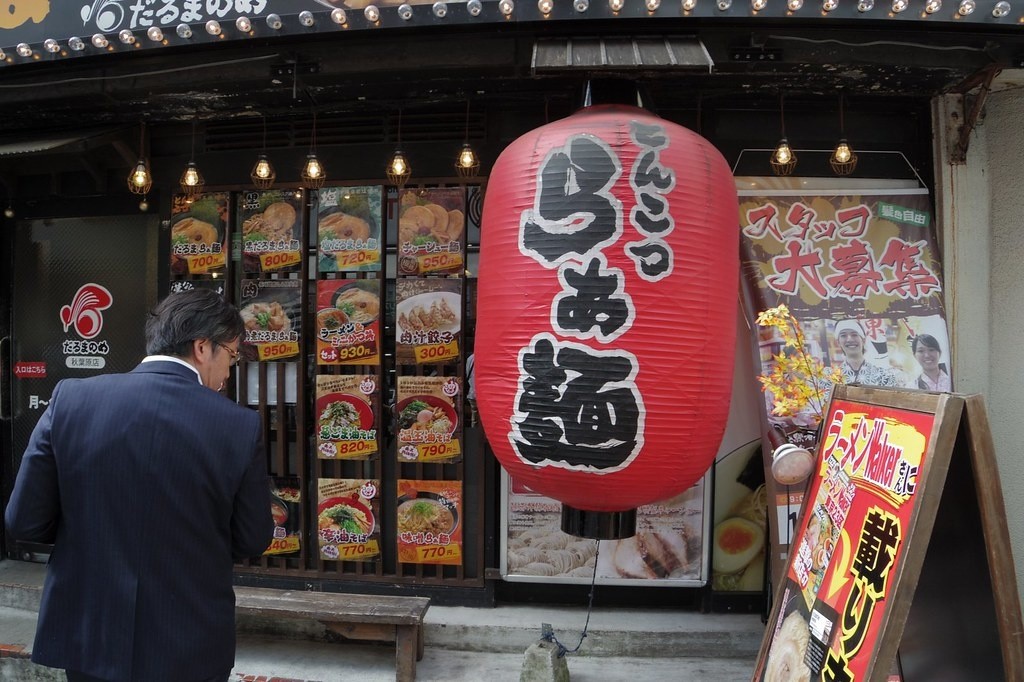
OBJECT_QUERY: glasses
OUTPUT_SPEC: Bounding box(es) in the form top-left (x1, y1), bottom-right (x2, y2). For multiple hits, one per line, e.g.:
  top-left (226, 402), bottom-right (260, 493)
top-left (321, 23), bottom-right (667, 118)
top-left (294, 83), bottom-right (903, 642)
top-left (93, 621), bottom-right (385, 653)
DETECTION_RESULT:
top-left (214, 340), bottom-right (241, 366)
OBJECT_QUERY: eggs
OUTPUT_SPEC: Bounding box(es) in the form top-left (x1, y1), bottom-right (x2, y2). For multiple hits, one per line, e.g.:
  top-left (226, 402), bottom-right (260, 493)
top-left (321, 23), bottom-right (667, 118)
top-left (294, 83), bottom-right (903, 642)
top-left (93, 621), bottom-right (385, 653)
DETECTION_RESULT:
top-left (712, 517), bottom-right (765, 573)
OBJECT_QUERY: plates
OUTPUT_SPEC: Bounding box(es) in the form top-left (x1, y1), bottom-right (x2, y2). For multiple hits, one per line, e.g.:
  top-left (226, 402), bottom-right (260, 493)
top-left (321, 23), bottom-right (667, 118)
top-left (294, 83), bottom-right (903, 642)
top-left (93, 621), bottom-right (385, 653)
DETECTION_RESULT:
top-left (331, 282), bottom-right (380, 326)
top-left (242, 207), bottom-right (299, 257)
top-left (319, 206), bottom-right (378, 256)
top-left (241, 297), bottom-right (295, 342)
top-left (171, 212), bottom-right (225, 260)
top-left (317, 308), bottom-right (350, 342)
top-left (396, 292), bottom-right (461, 343)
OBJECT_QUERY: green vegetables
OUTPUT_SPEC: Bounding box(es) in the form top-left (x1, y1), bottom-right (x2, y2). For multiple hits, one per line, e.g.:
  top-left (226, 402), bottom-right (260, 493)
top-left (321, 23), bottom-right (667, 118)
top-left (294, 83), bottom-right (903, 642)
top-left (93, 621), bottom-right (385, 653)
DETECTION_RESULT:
top-left (242, 231), bottom-right (268, 243)
top-left (319, 401), bottom-right (360, 425)
top-left (191, 193), bottom-right (433, 222)
top-left (399, 400), bottom-right (427, 429)
top-left (336, 299), bottom-right (355, 317)
top-left (411, 233), bottom-right (437, 247)
top-left (319, 228), bottom-right (337, 243)
top-left (171, 232), bottom-right (189, 252)
top-left (324, 505), bottom-right (364, 534)
top-left (324, 315), bottom-right (340, 330)
top-left (255, 311), bottom-right (273, 328)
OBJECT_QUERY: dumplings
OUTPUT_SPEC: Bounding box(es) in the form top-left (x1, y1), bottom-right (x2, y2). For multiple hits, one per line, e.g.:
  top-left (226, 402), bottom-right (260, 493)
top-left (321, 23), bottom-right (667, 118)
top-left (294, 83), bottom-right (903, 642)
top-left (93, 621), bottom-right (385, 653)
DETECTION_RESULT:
top-left (398, 297), bottom-right (455, 333)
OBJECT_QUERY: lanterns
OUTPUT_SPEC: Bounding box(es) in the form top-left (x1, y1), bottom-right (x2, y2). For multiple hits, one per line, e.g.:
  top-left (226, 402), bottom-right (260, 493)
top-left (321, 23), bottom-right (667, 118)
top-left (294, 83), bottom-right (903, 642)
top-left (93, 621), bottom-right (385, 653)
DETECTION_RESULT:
top-left (475, 99), bottom-right (742, 540)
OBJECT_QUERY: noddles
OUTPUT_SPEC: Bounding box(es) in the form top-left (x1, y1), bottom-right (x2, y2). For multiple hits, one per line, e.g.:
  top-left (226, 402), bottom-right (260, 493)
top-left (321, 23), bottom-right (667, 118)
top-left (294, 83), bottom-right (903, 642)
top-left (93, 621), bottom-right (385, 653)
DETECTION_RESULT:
top-left (711, 484), bottom-right (765, 591)
top-left (398, 498), bottom-right (454, 539)
top-left (336, 287), bottom-right (380, 322)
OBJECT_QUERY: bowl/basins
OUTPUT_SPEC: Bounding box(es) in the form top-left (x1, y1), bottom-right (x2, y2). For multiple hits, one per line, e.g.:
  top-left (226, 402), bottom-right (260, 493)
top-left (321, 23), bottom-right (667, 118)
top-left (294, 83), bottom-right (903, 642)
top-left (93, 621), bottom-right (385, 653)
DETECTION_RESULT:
top-left (318, 497), bottom-right (375, 538)
top-left (270, 494), bottom-right (289, 526)
top-left (397, 395), bottom-right (458, 440)
top-left (397, 491), bottom-right (459, 540)
top-left (317, 393), bottom-right (374, 441)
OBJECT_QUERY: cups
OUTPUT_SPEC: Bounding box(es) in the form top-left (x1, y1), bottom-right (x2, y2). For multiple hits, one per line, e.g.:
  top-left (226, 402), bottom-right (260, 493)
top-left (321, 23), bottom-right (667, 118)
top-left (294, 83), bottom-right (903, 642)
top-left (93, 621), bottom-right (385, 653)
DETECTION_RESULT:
top-left (870, 335), bottom-right (887, 356)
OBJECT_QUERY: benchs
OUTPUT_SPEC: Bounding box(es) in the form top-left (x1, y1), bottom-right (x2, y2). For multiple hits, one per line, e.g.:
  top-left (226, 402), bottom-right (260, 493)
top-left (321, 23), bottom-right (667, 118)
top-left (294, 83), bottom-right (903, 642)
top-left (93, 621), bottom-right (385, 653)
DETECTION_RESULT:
top-left (233, 584), bottom-right (436, 682)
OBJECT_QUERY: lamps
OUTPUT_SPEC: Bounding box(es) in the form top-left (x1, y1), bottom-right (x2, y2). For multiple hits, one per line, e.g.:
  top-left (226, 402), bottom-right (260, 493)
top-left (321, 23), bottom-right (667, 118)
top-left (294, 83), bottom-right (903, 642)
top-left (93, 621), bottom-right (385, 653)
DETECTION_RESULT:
top-left (127, 121), bottom-right (153, 197)
top-left (250, 116), bottom-right (277, 189)
top-left (301, 114), bottom-right (326, 191)
top-left (769, 95), bottom-right (797, 178)
top-left (180, 122), bottom-right (205, 194)
top-left (385, 108), bottom-right (412, 186)
top-left (829, 94), bottom-right (857, 176)
top-left (454, 100), bottom-right (482, 182)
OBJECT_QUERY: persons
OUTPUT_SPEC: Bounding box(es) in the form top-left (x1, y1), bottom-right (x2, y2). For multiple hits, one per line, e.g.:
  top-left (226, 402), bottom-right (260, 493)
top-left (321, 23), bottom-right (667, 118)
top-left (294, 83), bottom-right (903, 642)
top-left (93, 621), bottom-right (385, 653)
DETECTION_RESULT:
top-left (820, 320), bottom-right (901, 397)
top-left (910, 334), bottom-right (949, 393)
top-left (5, 289), bottom-right (275, 681)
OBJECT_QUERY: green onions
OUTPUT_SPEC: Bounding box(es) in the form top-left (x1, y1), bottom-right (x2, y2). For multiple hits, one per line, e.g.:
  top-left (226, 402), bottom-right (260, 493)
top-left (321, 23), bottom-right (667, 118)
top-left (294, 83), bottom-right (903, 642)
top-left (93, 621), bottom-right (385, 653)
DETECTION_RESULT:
top-left (412, 502), bottom-right (434, 517)
